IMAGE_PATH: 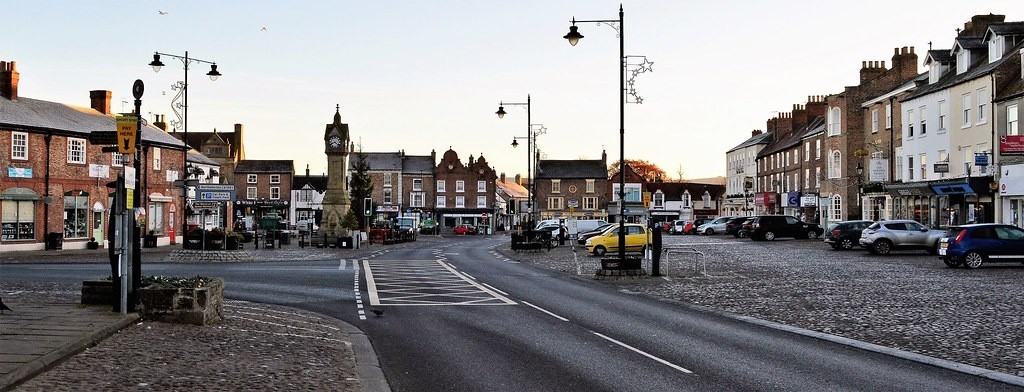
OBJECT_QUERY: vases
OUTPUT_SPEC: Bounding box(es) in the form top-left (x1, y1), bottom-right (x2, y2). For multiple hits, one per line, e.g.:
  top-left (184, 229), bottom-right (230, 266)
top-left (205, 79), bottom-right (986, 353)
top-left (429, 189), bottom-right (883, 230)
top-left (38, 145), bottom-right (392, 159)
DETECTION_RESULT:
top-left (81, 278), bottom-right (224, 326)
top-left (189, 232), bottom-right (223, 237)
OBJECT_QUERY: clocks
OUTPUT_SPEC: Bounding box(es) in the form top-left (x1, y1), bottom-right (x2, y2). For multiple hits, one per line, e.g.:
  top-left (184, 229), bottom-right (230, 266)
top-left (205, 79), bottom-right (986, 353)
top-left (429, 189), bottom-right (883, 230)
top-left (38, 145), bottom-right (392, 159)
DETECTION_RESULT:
top-left (328, 136), bottom-right (342, 150)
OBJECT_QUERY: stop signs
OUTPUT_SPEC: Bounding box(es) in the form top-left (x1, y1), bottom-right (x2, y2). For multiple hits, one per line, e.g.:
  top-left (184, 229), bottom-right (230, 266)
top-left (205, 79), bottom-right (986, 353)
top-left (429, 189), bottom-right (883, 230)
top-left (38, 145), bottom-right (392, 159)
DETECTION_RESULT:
top-left (481, 213), bottom-right (487, 219)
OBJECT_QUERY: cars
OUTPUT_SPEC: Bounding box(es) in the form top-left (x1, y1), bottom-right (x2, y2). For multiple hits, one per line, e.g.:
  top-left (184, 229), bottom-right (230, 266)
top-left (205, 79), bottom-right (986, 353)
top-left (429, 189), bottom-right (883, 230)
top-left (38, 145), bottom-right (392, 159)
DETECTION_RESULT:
top-left (578, 223), bottom-right (653, 256)
top-left (453, 224), bottom-right (476, 235)
top-left (535, 226), bottom-right (568, 241)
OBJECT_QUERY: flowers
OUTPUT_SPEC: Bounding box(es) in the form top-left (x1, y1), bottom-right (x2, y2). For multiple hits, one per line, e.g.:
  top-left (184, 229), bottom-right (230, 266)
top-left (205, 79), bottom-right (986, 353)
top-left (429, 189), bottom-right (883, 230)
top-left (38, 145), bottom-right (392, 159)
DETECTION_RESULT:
top-left (101, 273), bottom-right (215, 290)
top-left (190, 226), bottom-right (221, 232)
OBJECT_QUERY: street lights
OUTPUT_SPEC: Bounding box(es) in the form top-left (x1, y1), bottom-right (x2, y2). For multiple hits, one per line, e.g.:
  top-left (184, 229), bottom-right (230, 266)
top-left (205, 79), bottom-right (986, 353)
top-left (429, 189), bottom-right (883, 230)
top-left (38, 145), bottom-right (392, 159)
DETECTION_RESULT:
top-left (855, 163), bottom-right (864, 219)
top-left (148, 50), bottom-right (222, 248)
top-left (745, 188), bottom-right (749, 215)
top-left (562, 3), bottom-right (655, 257)
top-left (495, 93), bottom-right (547, 243)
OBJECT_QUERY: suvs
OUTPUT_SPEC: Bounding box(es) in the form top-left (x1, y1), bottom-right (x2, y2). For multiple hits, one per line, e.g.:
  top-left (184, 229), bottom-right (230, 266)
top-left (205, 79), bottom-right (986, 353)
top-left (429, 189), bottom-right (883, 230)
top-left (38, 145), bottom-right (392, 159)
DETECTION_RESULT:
top-left (824, 219), bottom-right (876, 250)
top-left (938, 223), bottom-right (1024, 269)
top-left (670, 215), bottom-right (754, 238)
top-left (374, 217), bottom-right (417, 240)
top-left (859, 219), bottom-right (946, 255)
top-left (297, 220), bottom-right (319, 234)
top-left (742, 215), bottom-right (825, 241)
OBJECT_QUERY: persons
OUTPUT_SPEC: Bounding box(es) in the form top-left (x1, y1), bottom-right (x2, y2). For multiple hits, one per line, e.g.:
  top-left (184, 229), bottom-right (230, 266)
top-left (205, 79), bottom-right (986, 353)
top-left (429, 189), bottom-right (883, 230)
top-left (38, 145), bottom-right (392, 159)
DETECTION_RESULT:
top-left (233, 219), bottom-right (243, 233)
top-left (522, 215), bottom-right (526, 229)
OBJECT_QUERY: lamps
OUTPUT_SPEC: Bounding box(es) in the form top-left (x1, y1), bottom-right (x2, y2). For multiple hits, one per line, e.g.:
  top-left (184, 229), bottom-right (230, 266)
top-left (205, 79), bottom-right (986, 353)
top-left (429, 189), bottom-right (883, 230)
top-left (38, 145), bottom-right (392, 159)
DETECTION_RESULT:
top-left (854, 141), bottom-right (890, 159)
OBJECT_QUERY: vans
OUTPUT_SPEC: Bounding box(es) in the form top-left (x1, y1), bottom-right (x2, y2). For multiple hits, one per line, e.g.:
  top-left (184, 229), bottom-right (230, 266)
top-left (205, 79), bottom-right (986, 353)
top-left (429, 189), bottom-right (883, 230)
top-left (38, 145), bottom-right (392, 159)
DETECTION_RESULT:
top-left (536, 219), bottom-right (609, 239)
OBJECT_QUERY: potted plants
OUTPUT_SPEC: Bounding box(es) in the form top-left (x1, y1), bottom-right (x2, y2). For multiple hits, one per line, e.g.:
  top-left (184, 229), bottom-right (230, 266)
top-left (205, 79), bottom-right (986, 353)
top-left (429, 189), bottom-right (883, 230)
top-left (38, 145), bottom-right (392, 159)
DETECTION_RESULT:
top-left (224, 230), bottom-right (244, 250)
top-left (88, 237), bottom-right (98, 250)
top-left (143, 230), bottom-right (158, 248)
top-left (337, 208), bottom-right (359, 249)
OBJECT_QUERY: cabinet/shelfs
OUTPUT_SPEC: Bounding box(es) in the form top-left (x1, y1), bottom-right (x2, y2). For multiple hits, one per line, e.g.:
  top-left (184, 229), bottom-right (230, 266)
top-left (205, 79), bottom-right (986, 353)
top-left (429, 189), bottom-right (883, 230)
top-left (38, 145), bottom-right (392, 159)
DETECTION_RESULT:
top-left (2, 228), bottom-right (33, 235)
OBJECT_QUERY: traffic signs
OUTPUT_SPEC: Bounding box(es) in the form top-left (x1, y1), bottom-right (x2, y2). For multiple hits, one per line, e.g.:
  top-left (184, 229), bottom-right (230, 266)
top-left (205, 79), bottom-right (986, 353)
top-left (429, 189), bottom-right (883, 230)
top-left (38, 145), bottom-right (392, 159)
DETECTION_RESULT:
top-left (89, 116), bottom-right (138, 154)
top-left (173, 179), bottom-right (199, 197)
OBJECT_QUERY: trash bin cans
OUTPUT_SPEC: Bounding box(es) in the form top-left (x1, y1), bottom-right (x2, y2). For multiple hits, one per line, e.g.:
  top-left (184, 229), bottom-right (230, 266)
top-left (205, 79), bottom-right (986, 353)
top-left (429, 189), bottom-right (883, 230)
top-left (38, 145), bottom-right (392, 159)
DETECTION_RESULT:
top-left (48, 232), bottom-right (63, 250)
top-left (280, 231), bottom-right (292, 244)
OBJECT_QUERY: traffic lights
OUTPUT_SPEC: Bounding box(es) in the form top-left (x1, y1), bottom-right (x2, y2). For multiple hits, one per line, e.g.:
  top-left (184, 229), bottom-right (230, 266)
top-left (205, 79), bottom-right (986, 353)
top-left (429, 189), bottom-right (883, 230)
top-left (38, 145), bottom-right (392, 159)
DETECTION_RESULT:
top-left (364, 198), bottom-right (372, 216)
top-left (506, 198), bottom-right (515, 215)
